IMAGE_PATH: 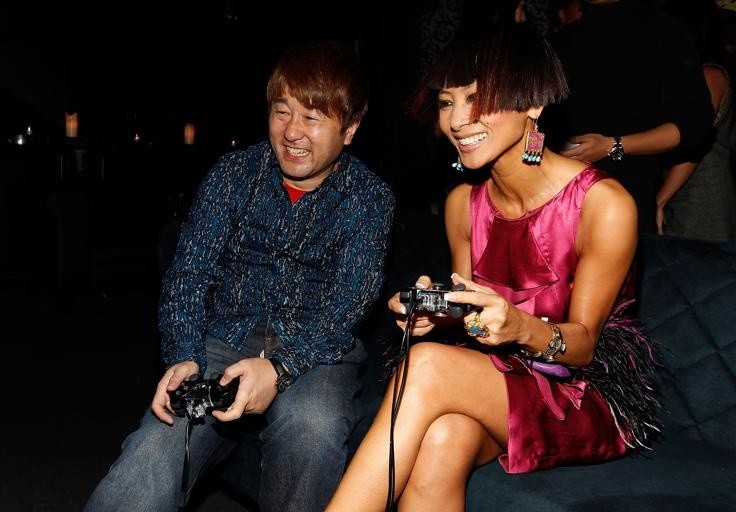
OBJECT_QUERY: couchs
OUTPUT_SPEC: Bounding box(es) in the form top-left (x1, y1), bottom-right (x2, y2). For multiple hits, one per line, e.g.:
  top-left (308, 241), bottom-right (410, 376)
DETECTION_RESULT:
top-left (219, 232), bottom-right (736, 512)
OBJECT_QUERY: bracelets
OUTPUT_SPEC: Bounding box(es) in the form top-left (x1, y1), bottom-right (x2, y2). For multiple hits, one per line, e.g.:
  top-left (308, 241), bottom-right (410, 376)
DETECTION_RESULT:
top-left (519, 321), bottom-right (567, 362)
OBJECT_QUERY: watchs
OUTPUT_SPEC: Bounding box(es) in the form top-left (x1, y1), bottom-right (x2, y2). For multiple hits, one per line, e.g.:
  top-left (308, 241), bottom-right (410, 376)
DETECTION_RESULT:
top-left (270, 355), bottom-right (293, 393)
top-left (606, 134), bottom-right (626, 164)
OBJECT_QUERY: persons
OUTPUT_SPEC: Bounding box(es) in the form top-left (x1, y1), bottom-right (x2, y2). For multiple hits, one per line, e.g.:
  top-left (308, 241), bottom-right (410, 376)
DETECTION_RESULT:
top-left (513, 1), bottom-right (712, 169)
top-left (324, 17), bottom-right (667, 511)
top-left (655, 52), bottom-right (736, 244)
top-left (77, 42), bottom-right (398, 511)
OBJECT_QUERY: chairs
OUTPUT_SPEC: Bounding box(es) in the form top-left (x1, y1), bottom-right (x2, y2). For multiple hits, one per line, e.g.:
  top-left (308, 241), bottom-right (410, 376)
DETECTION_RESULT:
top-left (26, 180), bottom-right (170, 313)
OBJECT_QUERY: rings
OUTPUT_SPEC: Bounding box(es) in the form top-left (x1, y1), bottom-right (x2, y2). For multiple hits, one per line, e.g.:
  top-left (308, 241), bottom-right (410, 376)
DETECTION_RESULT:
top-left (464, 314), bottom-right (490, 338)
